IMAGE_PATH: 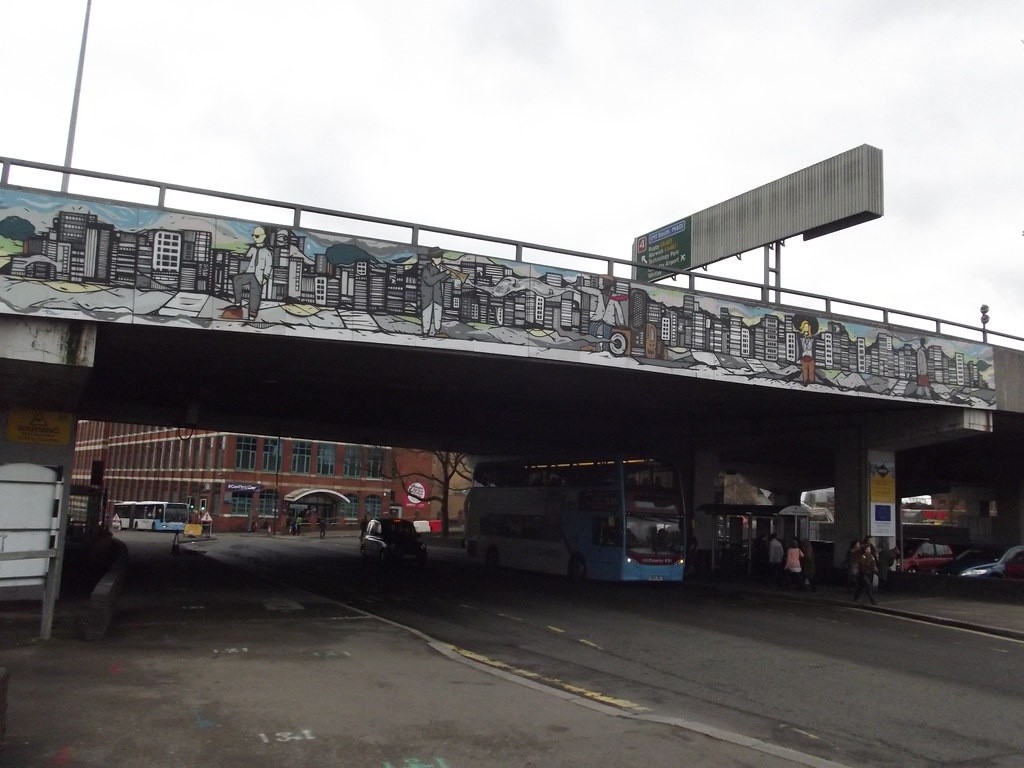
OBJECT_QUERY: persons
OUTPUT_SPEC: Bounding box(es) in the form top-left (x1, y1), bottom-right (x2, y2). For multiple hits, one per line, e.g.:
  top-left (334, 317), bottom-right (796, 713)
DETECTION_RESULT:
top-left (290, 515), bottom-right (302, 535)
top-left (359, 516), bottom-right (368, 539)
top-left (844, 536), bottom-right (889, 604)
top-left (190, 509), bottom-right (198, 523)
top-left (755, 531), bottom-right (817, 592)
top-left (319, 519), bottom-right (325, 539)
top-left (655, 476), bottom-right (661, 486)
top-left (686, 529), bottom-right (700, 576)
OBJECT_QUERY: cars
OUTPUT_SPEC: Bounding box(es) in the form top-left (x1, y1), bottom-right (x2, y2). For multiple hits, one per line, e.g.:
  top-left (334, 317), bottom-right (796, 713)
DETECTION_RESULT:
top-left (898, 535), bottom-right (1023, 603)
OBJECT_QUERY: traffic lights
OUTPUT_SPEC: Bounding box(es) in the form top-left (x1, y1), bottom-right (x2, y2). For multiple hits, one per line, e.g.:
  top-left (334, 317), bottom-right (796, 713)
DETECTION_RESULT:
top-left (200, 498), bottom-right (207, 513)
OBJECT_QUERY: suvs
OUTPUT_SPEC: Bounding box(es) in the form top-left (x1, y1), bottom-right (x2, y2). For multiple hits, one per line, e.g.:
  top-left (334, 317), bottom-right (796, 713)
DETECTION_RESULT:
top-left (360, 516), bottom-right (428, 565)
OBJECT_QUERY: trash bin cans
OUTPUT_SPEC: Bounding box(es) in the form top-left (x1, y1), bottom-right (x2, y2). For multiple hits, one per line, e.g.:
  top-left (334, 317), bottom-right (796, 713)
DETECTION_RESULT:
top-left (429, 520), bottom-right (442, 533)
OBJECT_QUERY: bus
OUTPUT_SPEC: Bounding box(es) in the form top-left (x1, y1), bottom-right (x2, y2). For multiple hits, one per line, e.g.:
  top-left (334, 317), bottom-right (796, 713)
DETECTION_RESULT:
top-left (463, 452), bottom-right (686, 590)
top-left (111, 499), bottom-right (194, 533)
